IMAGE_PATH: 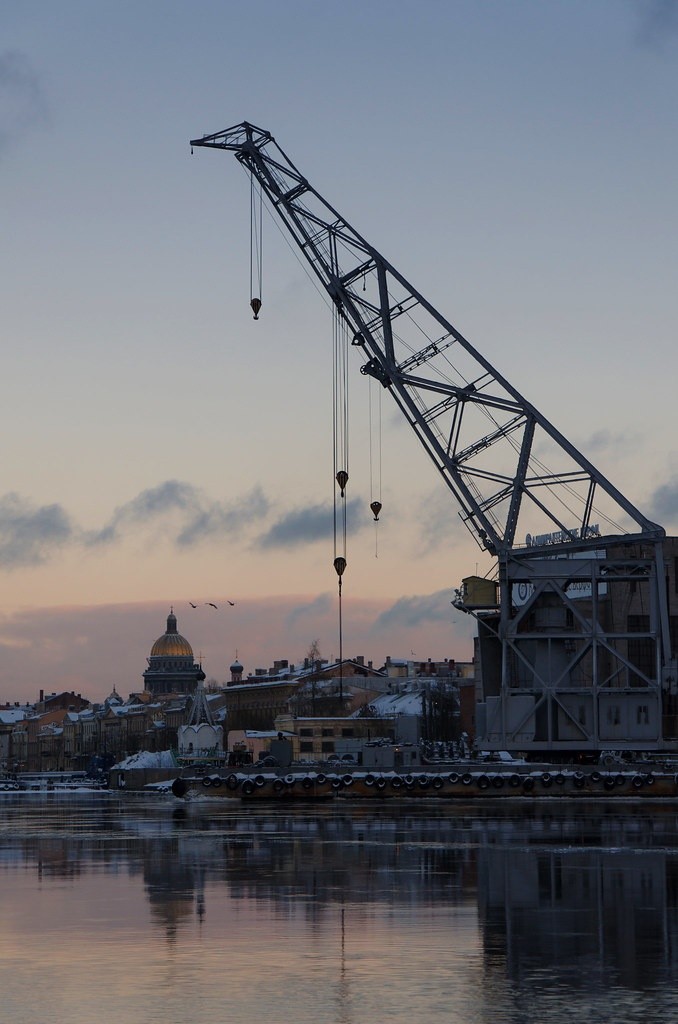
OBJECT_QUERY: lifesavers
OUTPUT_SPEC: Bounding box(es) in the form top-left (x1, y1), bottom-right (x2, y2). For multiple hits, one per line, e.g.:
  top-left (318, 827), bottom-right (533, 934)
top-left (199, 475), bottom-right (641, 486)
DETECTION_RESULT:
top-left (169, 772), bottom-right (678, 796)
